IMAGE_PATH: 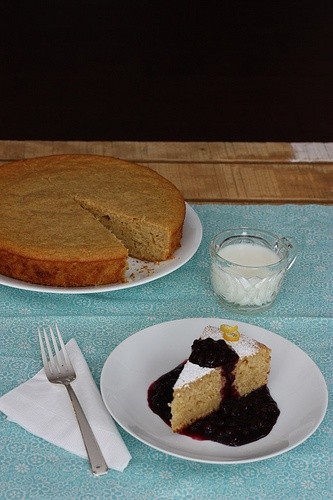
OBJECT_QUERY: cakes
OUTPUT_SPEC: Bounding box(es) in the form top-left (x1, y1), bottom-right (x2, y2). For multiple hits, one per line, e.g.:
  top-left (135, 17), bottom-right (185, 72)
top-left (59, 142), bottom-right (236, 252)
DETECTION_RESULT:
top-left (167, 323), bottom-right (272, 432)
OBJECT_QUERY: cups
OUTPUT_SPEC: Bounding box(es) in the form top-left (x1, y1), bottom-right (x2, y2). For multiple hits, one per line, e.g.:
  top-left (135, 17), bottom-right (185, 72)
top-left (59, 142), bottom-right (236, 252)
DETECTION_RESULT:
top-left (209, 227), bottom-right (300, 315)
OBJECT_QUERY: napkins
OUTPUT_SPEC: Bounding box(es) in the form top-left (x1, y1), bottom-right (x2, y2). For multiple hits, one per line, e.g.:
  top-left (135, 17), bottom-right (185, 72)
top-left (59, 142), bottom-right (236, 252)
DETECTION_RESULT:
top-left (0, 338), bottom-right (133, 472)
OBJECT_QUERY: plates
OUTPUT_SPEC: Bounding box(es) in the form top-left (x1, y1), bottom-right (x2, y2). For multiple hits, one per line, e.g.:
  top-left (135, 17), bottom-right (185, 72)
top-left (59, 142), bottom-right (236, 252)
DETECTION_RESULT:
top-left (0, 201), bottom-right (202, 294)
top-left (100, 318), bottom-right (328, 464)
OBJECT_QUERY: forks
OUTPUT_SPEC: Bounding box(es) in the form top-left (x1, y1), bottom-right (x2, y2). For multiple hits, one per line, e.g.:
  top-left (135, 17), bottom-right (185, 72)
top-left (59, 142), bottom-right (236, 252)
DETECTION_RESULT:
top-left (38, 324), bottom-right (108, 477)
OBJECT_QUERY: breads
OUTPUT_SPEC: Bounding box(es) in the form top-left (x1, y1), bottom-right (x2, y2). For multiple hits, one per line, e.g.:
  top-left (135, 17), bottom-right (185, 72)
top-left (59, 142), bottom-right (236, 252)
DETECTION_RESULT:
top-left (0, 153), bottom-right (186, 287)
